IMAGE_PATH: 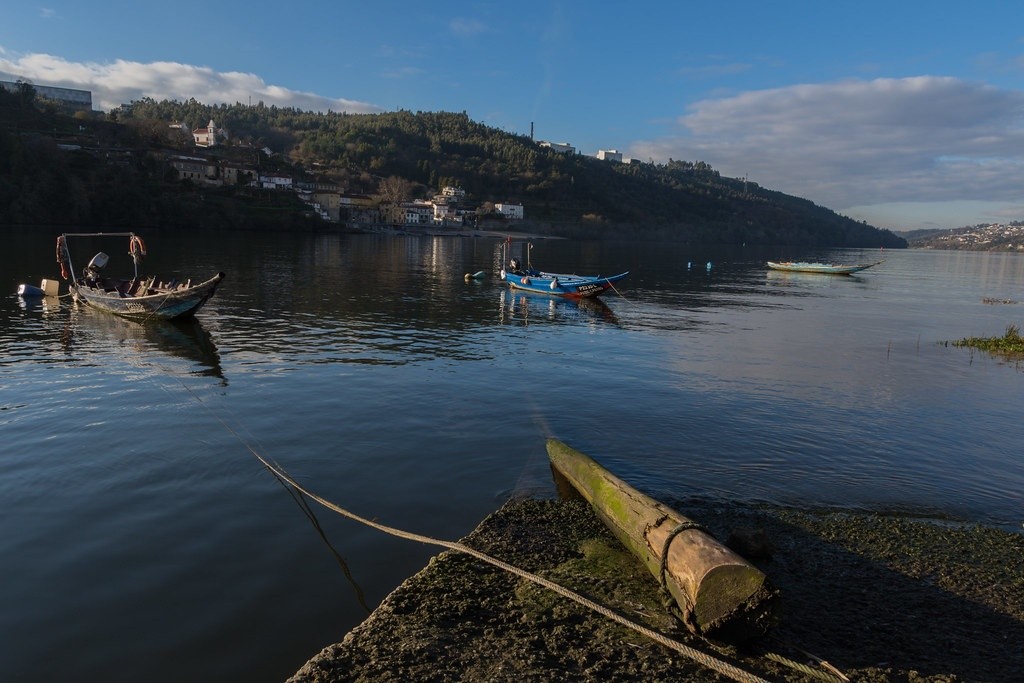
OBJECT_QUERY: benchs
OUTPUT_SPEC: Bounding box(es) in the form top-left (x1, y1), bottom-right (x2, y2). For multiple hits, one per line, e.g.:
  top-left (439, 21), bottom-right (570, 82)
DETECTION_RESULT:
top-left (546, 277), bottom-right (567, 279)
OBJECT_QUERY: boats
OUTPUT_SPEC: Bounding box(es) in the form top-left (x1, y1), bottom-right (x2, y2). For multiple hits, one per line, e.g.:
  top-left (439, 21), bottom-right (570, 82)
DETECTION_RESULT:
top-left (73, 253), bottom-right (224, 322)
top-left (499, 268), bottom-right (630, 298)
top-left (767, 260), bottom-right (886, 274)
